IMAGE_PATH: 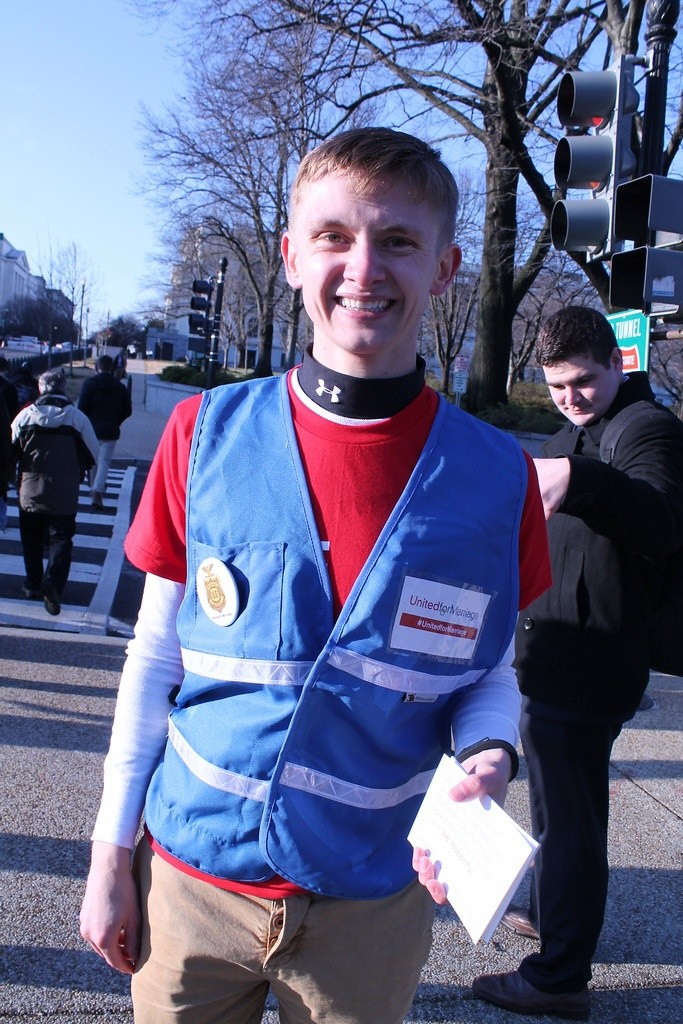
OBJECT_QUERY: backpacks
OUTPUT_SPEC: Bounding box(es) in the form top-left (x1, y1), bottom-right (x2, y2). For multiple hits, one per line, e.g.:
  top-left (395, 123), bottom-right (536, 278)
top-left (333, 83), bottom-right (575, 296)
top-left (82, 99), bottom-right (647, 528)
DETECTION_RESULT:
top-left (15, 381), bottom-right (34, 404)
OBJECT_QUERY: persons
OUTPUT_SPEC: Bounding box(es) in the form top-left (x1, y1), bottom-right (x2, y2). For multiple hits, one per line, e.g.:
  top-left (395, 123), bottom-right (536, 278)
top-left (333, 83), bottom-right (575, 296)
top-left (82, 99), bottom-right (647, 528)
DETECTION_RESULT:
top-left (77, 355), bottom-right (132, 511)
top-left (76, 129), bottom-right (562, 1024)
top-left (471, 307), bottom-right (683, 1019)
top-left (0, 356), bottom-right (42, 530)
top-left (11, 370), bottom-right (100, 615)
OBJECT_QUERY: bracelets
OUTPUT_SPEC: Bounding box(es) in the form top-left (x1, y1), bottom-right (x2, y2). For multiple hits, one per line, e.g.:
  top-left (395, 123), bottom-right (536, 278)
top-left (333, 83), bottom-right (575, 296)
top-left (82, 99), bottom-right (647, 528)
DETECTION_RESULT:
top-left (455, 737), bottom-right (519, 784)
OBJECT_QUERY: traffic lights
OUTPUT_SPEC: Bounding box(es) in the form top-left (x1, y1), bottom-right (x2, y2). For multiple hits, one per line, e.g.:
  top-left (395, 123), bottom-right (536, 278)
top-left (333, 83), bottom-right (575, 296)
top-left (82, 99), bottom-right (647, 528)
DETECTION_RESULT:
top-left (548, 54), bottom-right (639, 263)
top-left (188, 278), bottom-right (211, 328)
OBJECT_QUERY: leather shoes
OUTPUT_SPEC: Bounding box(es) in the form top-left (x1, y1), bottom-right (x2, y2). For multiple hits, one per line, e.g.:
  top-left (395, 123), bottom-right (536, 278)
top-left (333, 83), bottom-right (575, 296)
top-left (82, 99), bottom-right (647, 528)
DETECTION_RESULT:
top-left (500, 903), bottom-right (539, 940)
top-left (473, 970), bottom-right (590, 1020)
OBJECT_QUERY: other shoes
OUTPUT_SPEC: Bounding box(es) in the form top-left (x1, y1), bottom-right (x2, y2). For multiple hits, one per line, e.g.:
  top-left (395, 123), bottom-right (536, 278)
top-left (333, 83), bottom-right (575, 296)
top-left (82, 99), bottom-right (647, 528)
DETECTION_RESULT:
top-left (92, 493), bottom-right (103, 511)
top-left (22, 582), bottom-right (40, 599)
top-left (40, 579), bottom-right (60, 616)
